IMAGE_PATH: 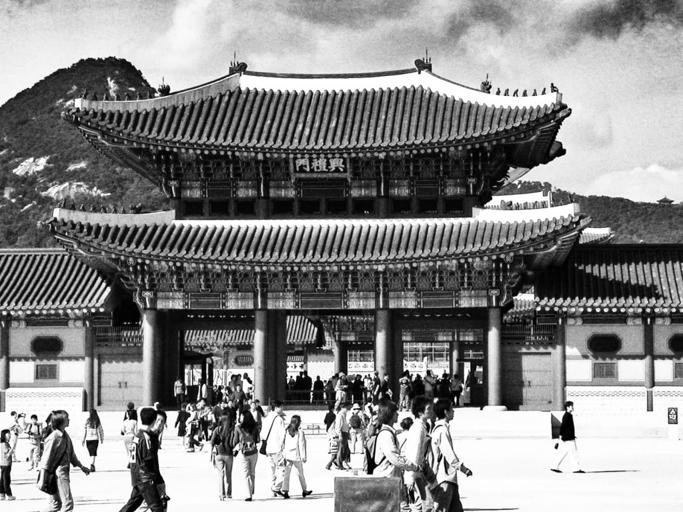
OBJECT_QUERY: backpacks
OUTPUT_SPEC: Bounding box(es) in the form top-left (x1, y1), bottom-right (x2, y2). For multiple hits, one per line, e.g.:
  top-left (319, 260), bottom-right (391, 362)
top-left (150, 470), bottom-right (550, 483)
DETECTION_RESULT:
top-left (242, 437), bottom-right (257, 456)
top-left (364, 429), bottom-right (396, 474)
top-left (349, 411), bottom-right (361, 428)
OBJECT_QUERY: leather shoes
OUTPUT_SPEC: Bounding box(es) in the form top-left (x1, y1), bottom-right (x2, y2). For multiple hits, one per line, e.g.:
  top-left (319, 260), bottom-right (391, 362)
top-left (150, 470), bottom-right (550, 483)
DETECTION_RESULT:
top-left (302, 491), bottom-right (312, 497)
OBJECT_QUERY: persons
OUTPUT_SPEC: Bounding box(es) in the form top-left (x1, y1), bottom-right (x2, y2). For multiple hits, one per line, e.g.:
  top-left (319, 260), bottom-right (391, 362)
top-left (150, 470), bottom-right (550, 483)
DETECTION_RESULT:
top-left (174, 377), bottom-right (188, 410)
top-left (550, 400), bottom-right (588, 475)
top-left (1, 408), bottom-right (104, 511)
top-left (326, 368), bottom-right (473, 510)
top-left (196, 370), bottom-right (323, 405)
top-left (120, 402), bottom-right (167, 511)
top-left (175, 398), bottom-right (312, 499)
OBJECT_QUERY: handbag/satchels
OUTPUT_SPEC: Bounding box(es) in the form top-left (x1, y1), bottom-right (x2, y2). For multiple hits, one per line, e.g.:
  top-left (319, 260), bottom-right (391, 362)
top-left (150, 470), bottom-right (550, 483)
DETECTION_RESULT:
top-left (260, 440), bottom-right (266, 454)
top-left (37, 471), bottom-right (58, 495)
